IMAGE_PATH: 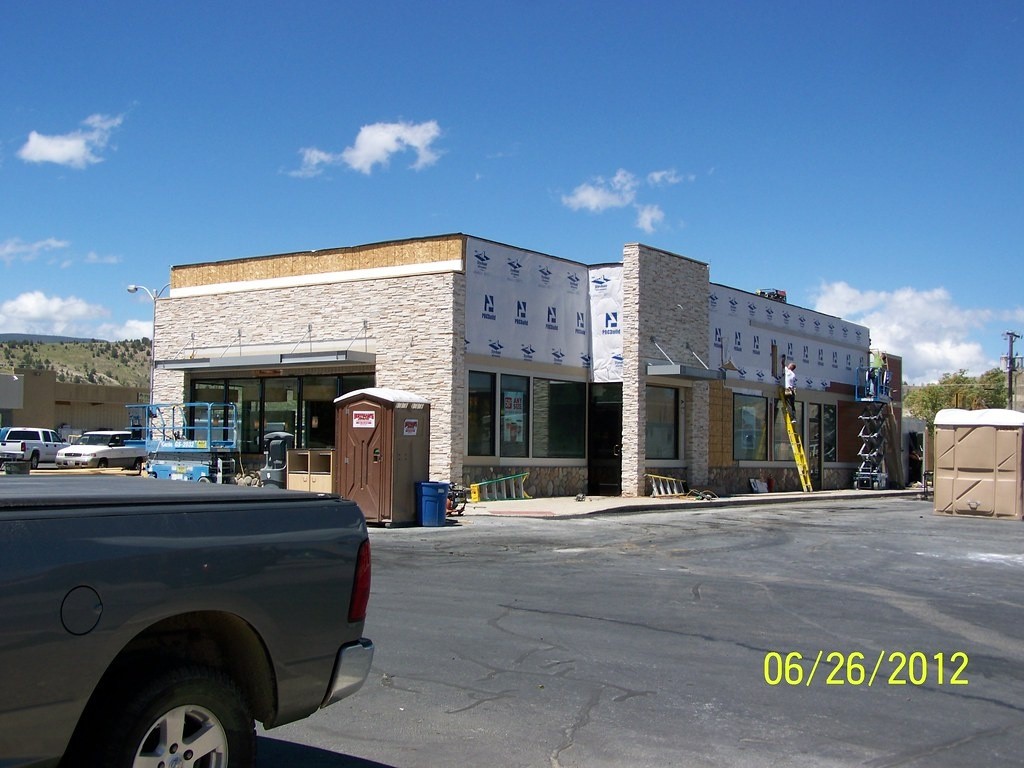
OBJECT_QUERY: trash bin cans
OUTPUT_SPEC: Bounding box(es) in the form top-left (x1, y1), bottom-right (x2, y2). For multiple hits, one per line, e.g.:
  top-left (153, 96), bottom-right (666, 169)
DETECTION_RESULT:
top-left (416, 480), bottom-right (450, 527)
top-left (260, 432), bottom-right (295, 490)
top-left (286, 447), bottom-right (336, 494)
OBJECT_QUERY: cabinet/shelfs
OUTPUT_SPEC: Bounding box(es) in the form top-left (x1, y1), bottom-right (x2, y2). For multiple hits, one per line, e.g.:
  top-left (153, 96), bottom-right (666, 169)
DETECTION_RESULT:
top-left (287, 448), bottom-right (336, 493)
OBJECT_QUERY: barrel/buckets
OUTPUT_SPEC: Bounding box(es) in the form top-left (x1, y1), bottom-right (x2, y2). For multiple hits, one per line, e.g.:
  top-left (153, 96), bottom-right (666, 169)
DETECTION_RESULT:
top-left (0, 461), bottom-right (31, 475)
top-left (416, 481), bottom-right (449, 526)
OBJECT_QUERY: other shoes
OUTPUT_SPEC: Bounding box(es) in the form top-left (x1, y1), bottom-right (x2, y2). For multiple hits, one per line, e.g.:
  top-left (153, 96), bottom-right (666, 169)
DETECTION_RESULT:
top-left (792, 418), bottom-right (796, 423)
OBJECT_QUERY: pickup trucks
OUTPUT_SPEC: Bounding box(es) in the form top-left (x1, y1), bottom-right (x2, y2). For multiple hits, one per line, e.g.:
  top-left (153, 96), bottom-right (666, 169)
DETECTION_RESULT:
top-left (0, 426), bottom-right (72, 470)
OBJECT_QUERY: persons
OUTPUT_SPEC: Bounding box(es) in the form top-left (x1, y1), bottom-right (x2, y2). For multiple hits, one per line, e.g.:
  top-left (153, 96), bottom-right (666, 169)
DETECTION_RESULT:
top-left (782, 354), bottom-right (796, 423)
top-left (871, 350), bottom-right (886, 397)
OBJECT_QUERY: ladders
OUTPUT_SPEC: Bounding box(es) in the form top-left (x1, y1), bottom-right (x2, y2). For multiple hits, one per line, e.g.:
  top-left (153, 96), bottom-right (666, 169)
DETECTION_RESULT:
top-left (644, 473), bottom-right (688, 498)
top-left (779, 386), bottom-right (815, 492)
top-left (469, 472), bottom-right (533, 503)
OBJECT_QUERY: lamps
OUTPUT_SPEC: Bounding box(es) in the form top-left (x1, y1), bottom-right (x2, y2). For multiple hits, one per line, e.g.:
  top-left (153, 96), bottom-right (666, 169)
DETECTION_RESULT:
top-left (213, 412), bottom-right (319, 431)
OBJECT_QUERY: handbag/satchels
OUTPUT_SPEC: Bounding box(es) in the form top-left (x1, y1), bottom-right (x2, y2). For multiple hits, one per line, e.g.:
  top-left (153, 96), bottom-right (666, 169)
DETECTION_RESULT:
top-left (785, 388), bottom-right (792, 395)
top-left (794, 388), bottom-right (796, 395)
top-left (870, 372), bottom-right (875, 378)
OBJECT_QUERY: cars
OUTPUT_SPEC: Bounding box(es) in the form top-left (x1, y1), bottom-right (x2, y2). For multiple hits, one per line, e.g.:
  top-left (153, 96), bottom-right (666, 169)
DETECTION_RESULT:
top-left (55, 430), bottom-right (146, 471)
top-left (1, 475), bottom-right (375, 768)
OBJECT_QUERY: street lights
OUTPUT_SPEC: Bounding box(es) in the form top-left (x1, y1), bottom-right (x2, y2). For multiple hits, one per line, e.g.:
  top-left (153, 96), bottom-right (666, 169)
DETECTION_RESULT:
top-left (127, 283), bottom-right (172, 426)
